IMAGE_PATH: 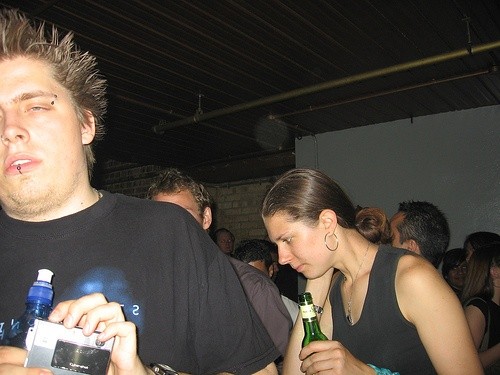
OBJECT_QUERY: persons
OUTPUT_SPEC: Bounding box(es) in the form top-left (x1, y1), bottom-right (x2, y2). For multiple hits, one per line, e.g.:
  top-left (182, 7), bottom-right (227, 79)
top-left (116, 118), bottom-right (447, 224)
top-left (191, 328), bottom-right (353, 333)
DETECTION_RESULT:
top-left (147, 169), bottom-right (294, 375)
top-left (262, 169), bottom-right (486, 375)
top-left (213, 200), bottom-right (500, 375)
top-left (0, 6), bottom-right (280, 375)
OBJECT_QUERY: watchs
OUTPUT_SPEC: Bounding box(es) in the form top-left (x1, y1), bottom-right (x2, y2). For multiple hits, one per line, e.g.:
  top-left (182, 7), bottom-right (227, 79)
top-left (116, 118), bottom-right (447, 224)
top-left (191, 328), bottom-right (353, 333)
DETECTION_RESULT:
top-left (147, 363), bottom-right (179, 375)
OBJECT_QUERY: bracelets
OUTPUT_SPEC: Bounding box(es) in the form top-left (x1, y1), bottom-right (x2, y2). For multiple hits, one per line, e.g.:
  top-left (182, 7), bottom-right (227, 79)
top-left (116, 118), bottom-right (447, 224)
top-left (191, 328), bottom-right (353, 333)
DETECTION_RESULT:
top-left (368, 363), bottom-right (399, 375)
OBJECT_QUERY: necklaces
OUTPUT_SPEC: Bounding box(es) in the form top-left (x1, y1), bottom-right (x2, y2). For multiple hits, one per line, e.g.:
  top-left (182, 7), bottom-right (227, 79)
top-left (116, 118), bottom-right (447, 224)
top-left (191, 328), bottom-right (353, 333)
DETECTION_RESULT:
top-left (343, 241), bottom-right (369, 325)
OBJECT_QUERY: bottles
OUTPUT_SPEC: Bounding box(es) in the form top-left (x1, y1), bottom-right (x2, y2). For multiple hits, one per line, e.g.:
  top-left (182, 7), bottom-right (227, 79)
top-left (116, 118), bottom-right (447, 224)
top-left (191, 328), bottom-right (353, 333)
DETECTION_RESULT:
top-left (2, 269), bottom-right (55, 350)
top-left (298, 292), bottom-right (329, 358)
